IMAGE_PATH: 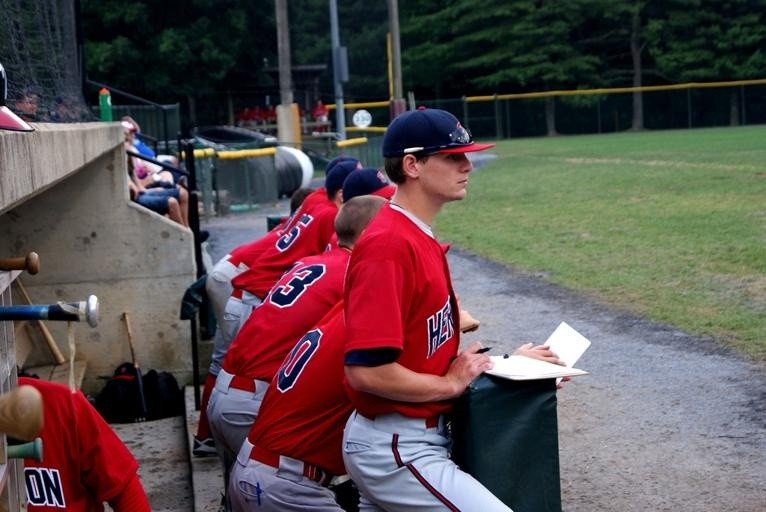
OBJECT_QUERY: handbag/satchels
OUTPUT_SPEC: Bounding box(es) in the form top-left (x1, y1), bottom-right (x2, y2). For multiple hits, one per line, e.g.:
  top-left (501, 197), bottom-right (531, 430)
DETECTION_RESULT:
top-left (94, 363), bottom-right (183, 423)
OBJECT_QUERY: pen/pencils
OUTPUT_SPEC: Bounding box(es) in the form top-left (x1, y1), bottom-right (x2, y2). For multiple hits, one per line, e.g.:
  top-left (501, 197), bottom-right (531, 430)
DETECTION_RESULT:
top-left (449, 346), bottom-right (494, 362)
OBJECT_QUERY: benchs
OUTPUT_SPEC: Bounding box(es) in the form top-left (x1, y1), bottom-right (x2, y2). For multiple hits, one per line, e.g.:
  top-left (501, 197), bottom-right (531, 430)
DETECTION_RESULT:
top-left (10, 277), bottom-right (88, 395)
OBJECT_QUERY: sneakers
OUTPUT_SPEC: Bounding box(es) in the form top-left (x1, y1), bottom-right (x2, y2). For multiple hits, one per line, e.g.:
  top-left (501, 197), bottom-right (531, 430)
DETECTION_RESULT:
top-left (193, 437), bottom-right (218, 456)
top-left (200, 230), bottom-right (209, 241)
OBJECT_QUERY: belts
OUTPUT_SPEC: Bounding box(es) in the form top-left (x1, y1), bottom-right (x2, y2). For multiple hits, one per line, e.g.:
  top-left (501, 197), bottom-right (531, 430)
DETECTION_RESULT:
top-left (231, 289), bottom-right (243, 300)
top-left (230, 376), bottom-right (257, 392)
top-left (357, 411), bottom-right (440, 429)
top-left (249, 446), bottom-right (320, 480)
top-left (226, 257), bottom-right (241, 267)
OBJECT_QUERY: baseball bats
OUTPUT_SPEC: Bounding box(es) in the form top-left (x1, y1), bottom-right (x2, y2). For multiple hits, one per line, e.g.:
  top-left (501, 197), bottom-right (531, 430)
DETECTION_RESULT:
top-left (15, 276), bottom-right (65, 365)
top-left (124, 312), bottom-right (148, 414)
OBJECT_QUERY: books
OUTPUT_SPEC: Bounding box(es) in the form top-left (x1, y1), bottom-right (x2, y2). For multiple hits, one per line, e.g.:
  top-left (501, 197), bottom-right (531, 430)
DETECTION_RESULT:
top-left (542, 322), bottom-right (592, 385)
top-left (483, 354), bottom-right (590, 384)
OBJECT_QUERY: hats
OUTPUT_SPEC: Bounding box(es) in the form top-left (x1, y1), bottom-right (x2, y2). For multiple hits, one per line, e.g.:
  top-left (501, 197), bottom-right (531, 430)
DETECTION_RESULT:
top-left (383, 106), bottom-right (495, 158)
top-left (122, 120), bottom-right (137, 132)
top-left (0, 66), bottom-right (35, 132)
top-left (326, 158), bottom-right (396, 203)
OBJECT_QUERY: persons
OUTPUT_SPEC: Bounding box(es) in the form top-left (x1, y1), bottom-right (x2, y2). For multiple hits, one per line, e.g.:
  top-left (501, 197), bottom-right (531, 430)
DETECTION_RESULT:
top-left (14, 376), bottom-right (154, 512)
top-left (188, 154), bottom-right (460, 489)
top-left (338, 102), bottom-right (516, 512)
top-left (311, 99), bottom-right (329, 133)
top-left (233, 102), bottom-right (310, 135)
top-left (223, 299), bottom-right (574, 510)
top-left (8, 85), bottom-right (209, 236)
